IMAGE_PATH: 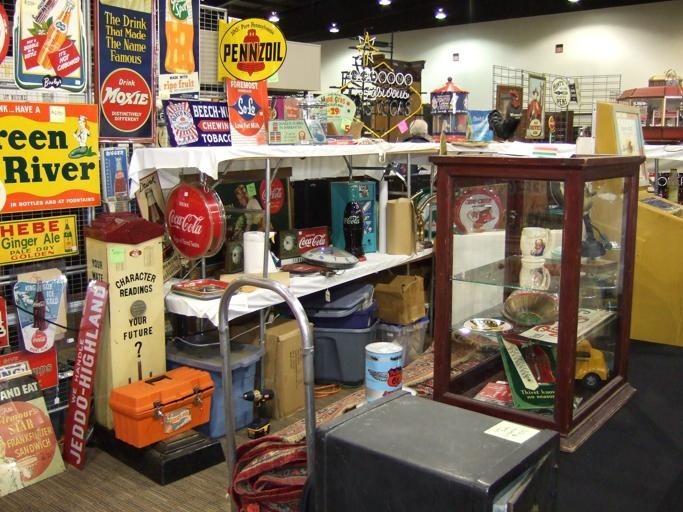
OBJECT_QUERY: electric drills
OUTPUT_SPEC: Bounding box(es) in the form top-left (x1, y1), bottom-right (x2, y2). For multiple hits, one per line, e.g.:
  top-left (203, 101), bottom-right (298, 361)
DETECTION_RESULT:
top-left (238, 387), bottom-right (274, 439)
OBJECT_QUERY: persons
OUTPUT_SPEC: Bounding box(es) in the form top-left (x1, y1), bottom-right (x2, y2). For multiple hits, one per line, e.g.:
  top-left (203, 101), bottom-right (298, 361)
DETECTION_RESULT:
top-left (233, 184), bottom-right (273, 233)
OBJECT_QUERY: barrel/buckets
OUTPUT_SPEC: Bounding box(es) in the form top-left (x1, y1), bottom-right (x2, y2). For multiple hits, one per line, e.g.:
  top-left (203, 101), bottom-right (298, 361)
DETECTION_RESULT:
top-left (430, 80), bottom-right (469, 143)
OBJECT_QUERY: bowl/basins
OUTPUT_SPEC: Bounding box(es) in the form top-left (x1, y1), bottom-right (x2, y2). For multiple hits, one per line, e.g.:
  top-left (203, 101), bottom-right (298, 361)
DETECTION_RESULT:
top-left (502, 291), bottom-right (558, 328)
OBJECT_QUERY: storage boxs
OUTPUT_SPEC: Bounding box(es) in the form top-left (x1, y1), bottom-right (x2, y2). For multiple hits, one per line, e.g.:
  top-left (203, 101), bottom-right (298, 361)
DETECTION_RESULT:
top-left (167, 275), bottom-right (431, 438)
top-left (107, 366), bottom-right (215, 450)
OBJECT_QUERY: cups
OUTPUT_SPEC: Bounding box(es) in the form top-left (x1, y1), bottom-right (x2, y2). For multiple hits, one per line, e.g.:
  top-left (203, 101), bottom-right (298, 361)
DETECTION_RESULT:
top-left (516, 258), bottom-right (553, 292)
top-left (518, 226), bottom-right (553, 262)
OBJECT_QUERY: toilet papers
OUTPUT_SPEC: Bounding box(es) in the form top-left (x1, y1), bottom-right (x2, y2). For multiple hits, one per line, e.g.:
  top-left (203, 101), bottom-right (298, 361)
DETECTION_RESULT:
top-left (242, 229), bottom-right (278, 274)
top-left (363, 339), bottom-right (404, 405)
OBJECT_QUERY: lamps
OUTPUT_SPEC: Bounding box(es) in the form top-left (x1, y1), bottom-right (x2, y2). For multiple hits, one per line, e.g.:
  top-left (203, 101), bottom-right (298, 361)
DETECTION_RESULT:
top-left (267, 11), bottom-right (279, 23)
top-left (328, 23), bottom-right (340, 34)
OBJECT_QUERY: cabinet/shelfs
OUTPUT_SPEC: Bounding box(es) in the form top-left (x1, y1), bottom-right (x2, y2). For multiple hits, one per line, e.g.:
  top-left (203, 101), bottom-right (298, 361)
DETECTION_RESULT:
top-left (425, 151), bottom-right (646, 454)
top-left (128, 136), bottom-right (445, 425)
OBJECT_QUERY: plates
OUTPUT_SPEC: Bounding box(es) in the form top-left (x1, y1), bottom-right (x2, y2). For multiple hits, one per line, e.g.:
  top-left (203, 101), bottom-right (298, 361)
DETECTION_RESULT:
top-left (463, 318), bottom-right (514, 332)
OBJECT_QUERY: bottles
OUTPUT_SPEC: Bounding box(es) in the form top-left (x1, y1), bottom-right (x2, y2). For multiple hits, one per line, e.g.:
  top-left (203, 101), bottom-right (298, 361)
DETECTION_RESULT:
top-left (145, 186), bottom-right (174, 262)
top-left (33, 280), bottom-right (47, 328)
top-left (342, 183), bottom-right (365, 258)
top-left (36, 2), bottom-right (73, 70)
top-left (409, 113), bottom-right (428, 137)
top-left (578, 125), bottom-right (591, 138)
top-left (245, 196), bottom-right (263, 231)
top-left (62, 219), bottom-right (74, 252)
top-left (114, 156), bottom-right (126, 202)
top-left (667, 168), bottom-right (679, 204)
top-left (648, 170), bottom-right (656, 193)
top-left (163, 0), bottom-right (195, 74)
